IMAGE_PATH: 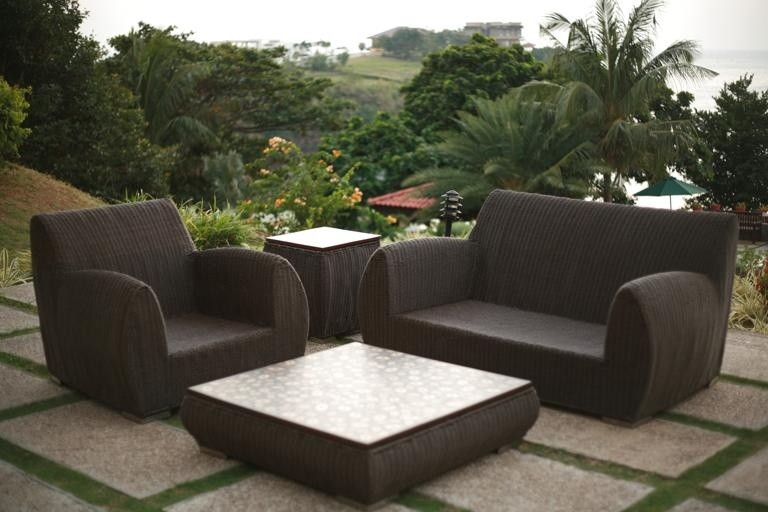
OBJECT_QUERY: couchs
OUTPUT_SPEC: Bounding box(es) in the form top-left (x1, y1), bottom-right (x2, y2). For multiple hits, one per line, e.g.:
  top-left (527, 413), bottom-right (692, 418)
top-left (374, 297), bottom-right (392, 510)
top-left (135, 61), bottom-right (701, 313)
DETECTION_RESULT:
top-left (356, 188), bottom-right (740, 424)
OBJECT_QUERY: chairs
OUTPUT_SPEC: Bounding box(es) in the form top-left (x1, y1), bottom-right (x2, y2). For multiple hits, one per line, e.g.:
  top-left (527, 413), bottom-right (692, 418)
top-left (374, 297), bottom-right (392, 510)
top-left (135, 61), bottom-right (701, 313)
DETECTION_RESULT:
top-left (28, 195), bottom-right (310, 421)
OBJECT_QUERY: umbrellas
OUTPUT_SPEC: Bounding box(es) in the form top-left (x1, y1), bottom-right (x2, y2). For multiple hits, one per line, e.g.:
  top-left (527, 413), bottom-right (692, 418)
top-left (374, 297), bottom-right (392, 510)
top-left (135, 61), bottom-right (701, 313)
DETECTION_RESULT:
top-left (632, 179), bottom-right (707, 209)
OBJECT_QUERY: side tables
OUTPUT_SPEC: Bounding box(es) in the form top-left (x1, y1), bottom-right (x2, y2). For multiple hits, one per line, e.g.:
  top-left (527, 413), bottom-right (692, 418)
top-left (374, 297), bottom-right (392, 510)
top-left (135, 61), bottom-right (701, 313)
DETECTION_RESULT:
top-left (263, 225), bottom-right (381, 340)
